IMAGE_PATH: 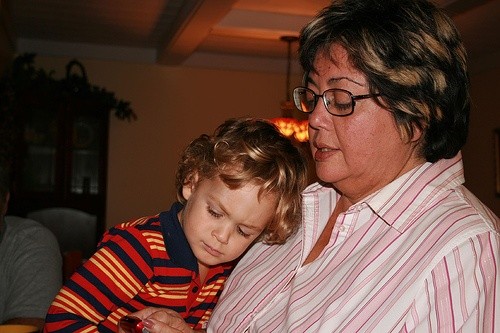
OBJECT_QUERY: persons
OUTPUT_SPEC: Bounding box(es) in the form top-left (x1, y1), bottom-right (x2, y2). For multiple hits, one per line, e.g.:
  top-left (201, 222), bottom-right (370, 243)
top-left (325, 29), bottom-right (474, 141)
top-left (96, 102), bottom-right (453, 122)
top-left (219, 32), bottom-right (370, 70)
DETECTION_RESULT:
top-left (116, 0), bottom-right (500, 333)
top-left (0, 166), bottom-right (63, 332)
top-left (25, 157), bottom-right (97, 255)
top-left (44, 118), bottom-right (307, 333)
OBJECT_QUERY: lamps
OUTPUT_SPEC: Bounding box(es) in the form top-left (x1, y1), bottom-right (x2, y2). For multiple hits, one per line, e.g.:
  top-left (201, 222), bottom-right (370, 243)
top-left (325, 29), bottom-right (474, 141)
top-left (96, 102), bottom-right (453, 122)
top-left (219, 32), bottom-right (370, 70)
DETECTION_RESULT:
top-left (265, 35), bottom-right (310, 142)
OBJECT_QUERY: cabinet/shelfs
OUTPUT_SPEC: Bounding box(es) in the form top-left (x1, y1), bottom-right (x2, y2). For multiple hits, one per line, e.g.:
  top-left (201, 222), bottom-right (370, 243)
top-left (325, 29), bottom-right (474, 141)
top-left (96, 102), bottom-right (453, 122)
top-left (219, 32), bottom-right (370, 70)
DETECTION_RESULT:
top-left (13, 93), bottom-right (108, 213)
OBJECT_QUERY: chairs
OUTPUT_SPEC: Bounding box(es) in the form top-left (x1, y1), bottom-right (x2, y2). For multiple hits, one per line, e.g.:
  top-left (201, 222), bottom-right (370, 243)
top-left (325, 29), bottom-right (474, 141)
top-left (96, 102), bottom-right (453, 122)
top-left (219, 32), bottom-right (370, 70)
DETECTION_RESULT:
top-left (27, 207), bottom-right (97, 261)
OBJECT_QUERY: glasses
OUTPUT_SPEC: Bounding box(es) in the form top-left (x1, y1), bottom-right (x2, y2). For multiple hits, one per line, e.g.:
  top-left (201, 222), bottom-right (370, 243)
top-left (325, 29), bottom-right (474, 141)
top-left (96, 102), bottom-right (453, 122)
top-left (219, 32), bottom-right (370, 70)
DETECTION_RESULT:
top-left (291, 87), bottom-right (383, 117)
